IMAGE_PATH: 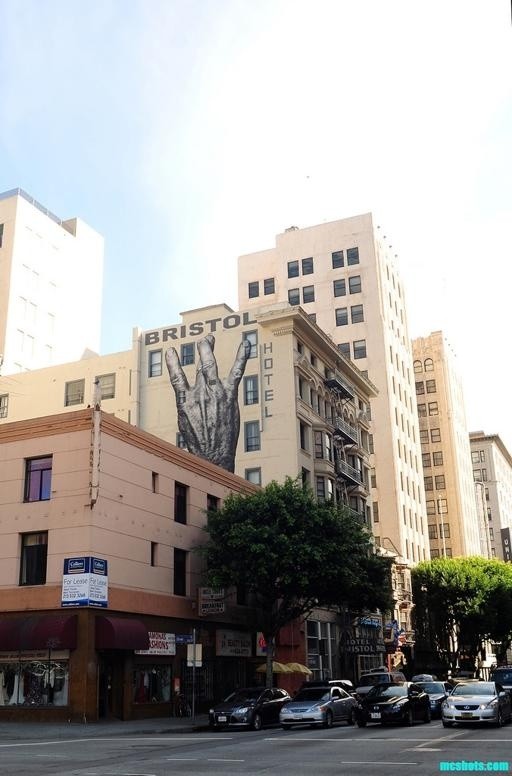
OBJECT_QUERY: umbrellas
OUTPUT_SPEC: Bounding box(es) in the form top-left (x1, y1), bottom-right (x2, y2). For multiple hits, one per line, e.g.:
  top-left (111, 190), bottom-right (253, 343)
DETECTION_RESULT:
top-left (285, 662), bottom-right (312, 675)
top-left (256, 661), bottom-right (294, 674)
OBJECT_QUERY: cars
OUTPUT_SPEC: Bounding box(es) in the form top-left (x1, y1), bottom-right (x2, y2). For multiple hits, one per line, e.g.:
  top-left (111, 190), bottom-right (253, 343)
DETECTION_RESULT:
top-left (208, 687), bottom-right (292, 732)
top-left (279, 687), bottom-right (359, 730)
top-left (356, 667), bottom-right (512, 728)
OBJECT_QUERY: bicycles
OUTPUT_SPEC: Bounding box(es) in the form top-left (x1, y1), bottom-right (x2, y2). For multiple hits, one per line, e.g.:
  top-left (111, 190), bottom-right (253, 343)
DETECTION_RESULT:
top-left (175, 693), bottom-right (192, 718)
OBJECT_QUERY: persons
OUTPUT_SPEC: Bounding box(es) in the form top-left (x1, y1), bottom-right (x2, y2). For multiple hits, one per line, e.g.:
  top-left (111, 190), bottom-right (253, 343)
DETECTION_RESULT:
top-left (164, 335), bottom-right (251, 474)
top-left (0, 665), bottom-right (55, 705)
top-left (172, 671), bottom-right (183, 717)
top-left (132, 666), bottom-right (172, 703)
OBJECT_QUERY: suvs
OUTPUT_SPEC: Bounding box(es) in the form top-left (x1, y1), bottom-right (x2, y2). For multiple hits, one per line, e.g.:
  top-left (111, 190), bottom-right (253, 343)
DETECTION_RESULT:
top-left (299, 679), bottom-right (359, 703)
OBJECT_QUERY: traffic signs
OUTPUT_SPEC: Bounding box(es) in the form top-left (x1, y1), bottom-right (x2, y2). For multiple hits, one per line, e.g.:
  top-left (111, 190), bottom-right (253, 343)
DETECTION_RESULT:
top-left (174, 635), bottom-right (193, 643)
